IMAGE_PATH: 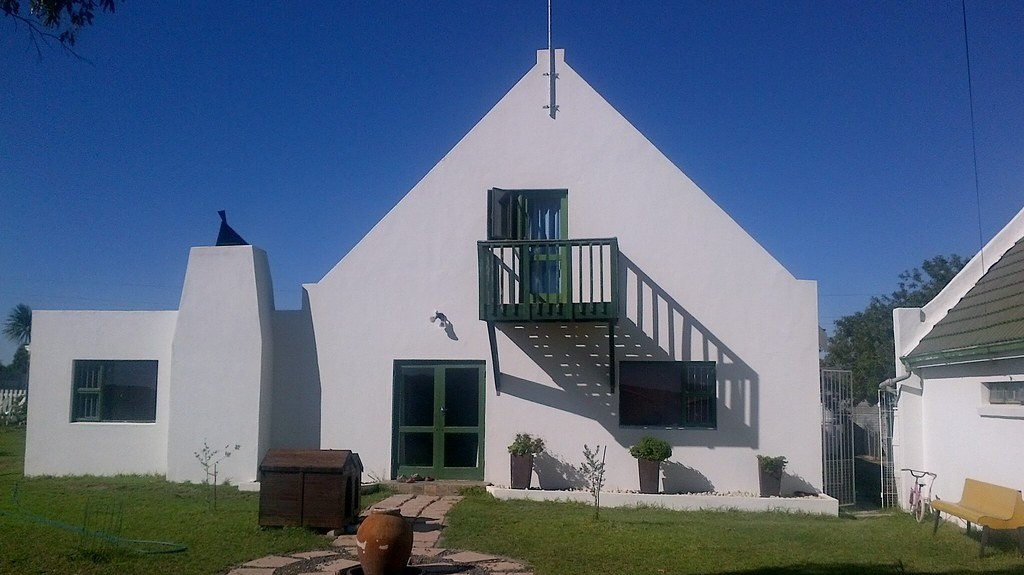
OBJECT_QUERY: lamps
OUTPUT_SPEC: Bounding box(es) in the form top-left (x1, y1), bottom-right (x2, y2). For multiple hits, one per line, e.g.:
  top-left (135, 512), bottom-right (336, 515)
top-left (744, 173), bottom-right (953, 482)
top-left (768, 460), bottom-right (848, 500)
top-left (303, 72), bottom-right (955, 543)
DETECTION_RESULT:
top-left (430, 312), bottom-right (445, 322)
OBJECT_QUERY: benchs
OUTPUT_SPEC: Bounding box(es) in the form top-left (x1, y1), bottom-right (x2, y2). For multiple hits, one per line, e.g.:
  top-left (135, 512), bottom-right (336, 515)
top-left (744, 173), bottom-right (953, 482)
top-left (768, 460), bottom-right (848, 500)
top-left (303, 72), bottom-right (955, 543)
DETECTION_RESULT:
top-left (931, 478), bottom-right (1024, 558)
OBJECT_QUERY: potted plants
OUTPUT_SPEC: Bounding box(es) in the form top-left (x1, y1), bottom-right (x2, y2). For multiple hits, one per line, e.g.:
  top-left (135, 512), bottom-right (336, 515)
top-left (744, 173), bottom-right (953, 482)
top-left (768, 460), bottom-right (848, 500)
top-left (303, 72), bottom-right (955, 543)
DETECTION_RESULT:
top-left (757, 455), bottom-right (784, 496)
top-left (507, 434), bottom-right (546, 490)
top-left (629, 434), bottom-right (673, 494)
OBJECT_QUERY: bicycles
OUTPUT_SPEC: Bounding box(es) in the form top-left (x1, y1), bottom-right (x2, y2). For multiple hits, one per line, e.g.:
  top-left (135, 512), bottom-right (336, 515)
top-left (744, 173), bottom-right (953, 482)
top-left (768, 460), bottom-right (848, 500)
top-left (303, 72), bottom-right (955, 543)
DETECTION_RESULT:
top-left (901, 468), bottom-right (933, 523)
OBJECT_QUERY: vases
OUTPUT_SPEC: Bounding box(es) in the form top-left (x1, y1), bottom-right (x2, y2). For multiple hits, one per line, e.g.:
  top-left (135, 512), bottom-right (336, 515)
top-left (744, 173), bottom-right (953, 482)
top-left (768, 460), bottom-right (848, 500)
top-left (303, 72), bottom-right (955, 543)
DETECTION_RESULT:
top-left (356, 507), bottom-right (412, 575)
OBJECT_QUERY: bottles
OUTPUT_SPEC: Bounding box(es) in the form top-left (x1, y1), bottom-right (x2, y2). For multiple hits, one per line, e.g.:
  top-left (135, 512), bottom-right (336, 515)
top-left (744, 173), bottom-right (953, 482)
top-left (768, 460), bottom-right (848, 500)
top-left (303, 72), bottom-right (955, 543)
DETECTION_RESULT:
top-left (356, 507), bottom-right (413, 575)
